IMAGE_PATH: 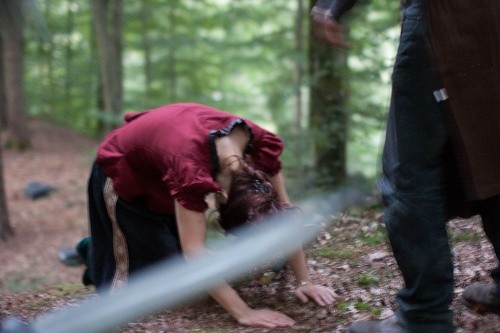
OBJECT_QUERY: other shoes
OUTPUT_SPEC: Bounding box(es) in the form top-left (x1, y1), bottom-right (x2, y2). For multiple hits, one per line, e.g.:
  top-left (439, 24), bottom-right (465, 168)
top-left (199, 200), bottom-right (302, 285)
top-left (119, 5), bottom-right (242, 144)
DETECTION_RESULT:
top-left (59, 249), bottom-right (82, 265)
top-left (350, 314), bottom-right (406, 333)
top-left (461, 284), bottom-right (500, 313)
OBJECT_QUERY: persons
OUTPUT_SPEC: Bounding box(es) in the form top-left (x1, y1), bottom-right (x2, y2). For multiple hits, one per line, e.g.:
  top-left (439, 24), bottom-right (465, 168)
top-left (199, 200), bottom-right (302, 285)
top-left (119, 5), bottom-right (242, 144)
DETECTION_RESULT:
top-left (310, 0), bottom-right (500, 333)
top-left (75, 103), bottom-right (338, 327)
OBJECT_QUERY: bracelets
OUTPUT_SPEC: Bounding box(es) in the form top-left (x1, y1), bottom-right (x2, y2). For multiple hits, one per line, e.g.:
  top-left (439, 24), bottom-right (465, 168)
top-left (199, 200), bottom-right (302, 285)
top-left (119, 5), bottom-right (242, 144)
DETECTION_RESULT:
top-left (299, 281), bottom-right (309, 285)
